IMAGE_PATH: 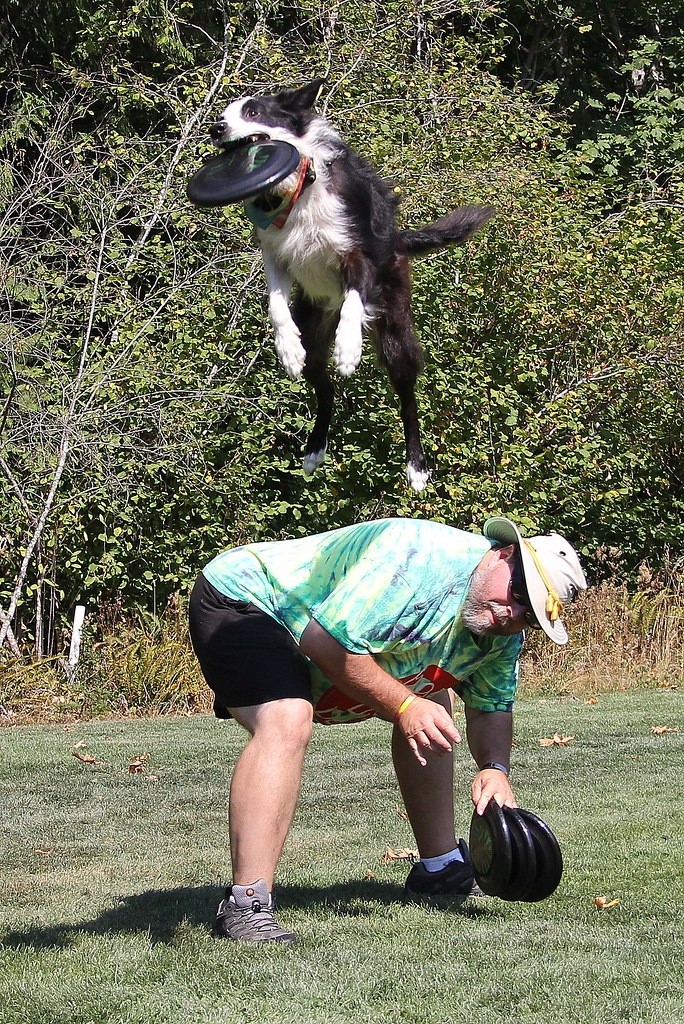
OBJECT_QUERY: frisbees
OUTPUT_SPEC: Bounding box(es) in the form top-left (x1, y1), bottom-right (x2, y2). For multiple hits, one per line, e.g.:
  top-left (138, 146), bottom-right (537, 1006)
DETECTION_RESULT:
top-left (514, 808), bottom-right (564, 902)
top-left (185, 139), bottom-right (300, 207)
top-left (468, 801), bottom-right (511, 897)
top-left (498, 806), bottom-right (535, 901)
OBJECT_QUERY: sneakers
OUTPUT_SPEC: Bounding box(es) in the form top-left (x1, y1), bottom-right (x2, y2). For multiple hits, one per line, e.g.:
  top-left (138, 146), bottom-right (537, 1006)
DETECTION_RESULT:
top-left (215, 878), bottom-right (298, 948)
top-left (405, 838), bottom-right (491, 900)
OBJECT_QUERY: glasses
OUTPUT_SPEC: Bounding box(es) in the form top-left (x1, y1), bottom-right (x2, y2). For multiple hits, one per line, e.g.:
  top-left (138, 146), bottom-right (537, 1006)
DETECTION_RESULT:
top-left (509, 556), bottom-right (542, 630)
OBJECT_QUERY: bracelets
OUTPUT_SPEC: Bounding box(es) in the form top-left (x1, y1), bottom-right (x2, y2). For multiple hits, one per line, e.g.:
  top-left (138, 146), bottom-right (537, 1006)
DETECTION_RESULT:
top-left (393, 694), bottom-right (416, 721)
top-left (480, 763), bottom-right (507, 776)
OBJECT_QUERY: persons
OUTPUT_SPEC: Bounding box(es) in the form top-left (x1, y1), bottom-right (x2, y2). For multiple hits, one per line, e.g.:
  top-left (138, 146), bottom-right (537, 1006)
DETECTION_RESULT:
top-left (190, 517), bottom-right (589, 945)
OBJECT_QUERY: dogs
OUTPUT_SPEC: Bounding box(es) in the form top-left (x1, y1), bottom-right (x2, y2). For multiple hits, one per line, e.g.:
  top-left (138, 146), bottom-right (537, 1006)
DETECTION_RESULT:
top-left (206, 77), bottom-right (498, 492)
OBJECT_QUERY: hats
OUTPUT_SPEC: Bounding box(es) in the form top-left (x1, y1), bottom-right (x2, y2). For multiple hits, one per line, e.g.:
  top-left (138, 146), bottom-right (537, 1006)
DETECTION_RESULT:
top-left (484, 517), bottom-right (587, 648)
